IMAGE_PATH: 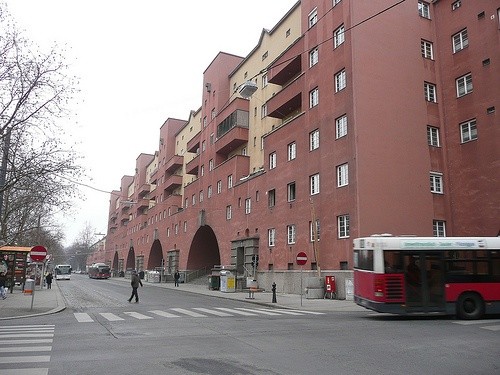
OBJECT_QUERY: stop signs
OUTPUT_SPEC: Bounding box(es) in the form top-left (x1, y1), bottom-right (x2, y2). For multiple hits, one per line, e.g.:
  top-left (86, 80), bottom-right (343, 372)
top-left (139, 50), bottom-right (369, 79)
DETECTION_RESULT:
top-left (296, 252), bottom-right (308, 265)
top-left (30, 246), bottom-right (47, 261)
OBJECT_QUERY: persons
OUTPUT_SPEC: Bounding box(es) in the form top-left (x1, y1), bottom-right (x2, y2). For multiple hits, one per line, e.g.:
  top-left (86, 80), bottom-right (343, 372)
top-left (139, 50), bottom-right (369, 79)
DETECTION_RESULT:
top-left (47, 273), bottom-right (52, 288)
top-left (174, 270), bottom-right (180, 287)
top-left (128, 270), bottom-right (143, 303)
top-left (0, 261), bottom-right (7, 300)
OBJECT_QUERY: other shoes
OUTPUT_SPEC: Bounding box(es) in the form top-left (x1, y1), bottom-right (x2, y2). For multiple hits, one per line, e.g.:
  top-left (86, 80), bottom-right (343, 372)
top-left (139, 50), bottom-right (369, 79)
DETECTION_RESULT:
top-left (3, 297), bottom-right (7, 300)
top-left (128, 300), bottom-right (131, 303)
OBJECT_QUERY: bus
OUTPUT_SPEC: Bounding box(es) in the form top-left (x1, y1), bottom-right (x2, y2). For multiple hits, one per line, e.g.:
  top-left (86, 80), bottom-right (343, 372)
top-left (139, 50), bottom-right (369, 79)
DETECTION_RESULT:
top-left (88, 263), bottom-right (111, 279)
top-left (54, 264), bottom-right (72, 280)
top-left (352, 233), bottom-right (500, 320)
